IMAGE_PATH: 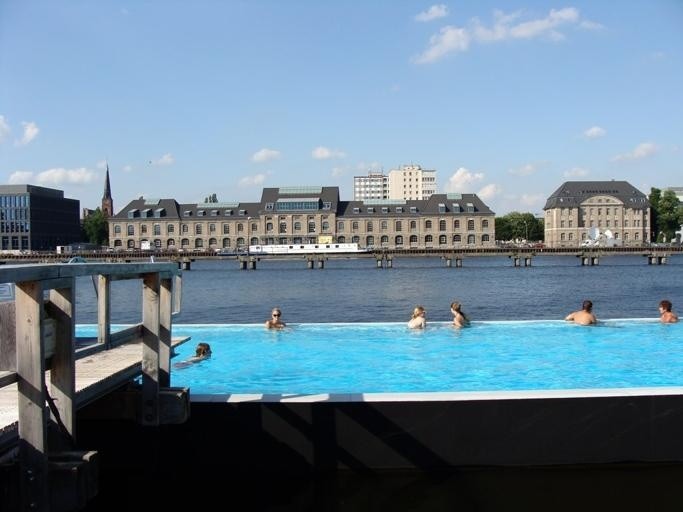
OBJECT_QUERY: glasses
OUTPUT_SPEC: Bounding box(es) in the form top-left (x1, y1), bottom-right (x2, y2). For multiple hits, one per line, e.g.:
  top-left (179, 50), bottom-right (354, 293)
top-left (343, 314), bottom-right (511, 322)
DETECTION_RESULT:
top-left (273, 314), bottom-right (280, 317)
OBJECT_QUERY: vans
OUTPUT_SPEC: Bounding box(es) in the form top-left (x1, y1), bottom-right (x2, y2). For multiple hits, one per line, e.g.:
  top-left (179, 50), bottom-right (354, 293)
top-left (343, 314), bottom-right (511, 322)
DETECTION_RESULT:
top-left (581, 240), bottom-right (599, 249)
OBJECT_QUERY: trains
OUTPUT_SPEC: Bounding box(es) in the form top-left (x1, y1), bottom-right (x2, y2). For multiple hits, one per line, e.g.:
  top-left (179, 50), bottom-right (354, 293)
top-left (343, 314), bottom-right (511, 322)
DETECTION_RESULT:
top-left (243, 242), bottom-right (362, 253)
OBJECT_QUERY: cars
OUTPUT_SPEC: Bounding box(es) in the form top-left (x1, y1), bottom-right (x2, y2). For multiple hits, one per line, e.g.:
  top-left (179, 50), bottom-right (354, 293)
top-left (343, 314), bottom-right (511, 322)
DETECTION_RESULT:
top-left (72, 243), bottom-right (248, 254)
top-left (528, 243), bottom-right (546, 248)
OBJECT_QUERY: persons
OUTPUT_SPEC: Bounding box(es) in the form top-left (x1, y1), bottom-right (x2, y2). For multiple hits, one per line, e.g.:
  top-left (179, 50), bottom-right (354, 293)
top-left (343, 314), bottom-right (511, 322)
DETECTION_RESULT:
top-left (174, 343), bottom-right (212, 370)
top-left (265, 308), bottom-right (286, 330)
top-left (565, 300), bottom-right (595, 325)
top-left (451, 301), bottom-right (470, 328)
top-left (408, 306), bottom-right (426, 329)
top-left (659, 300), bottom-right (678, 323)
